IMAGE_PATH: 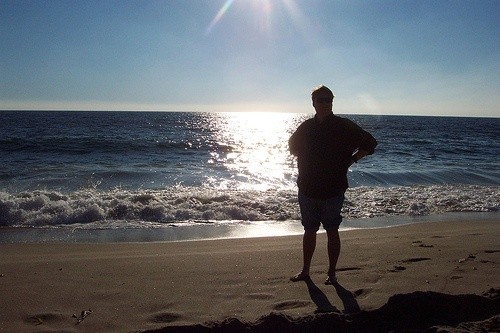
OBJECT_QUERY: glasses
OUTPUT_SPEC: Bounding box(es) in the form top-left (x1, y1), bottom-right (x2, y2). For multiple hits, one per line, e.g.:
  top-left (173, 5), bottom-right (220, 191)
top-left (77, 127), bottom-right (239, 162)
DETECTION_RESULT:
top-left (316, 98), bottom-right (332, 103)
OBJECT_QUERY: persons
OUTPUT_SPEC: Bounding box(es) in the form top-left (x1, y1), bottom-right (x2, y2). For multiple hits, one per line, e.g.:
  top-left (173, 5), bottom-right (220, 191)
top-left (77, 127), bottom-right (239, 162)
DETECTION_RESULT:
top-left (287, 85), bottom-right (378, 286)
